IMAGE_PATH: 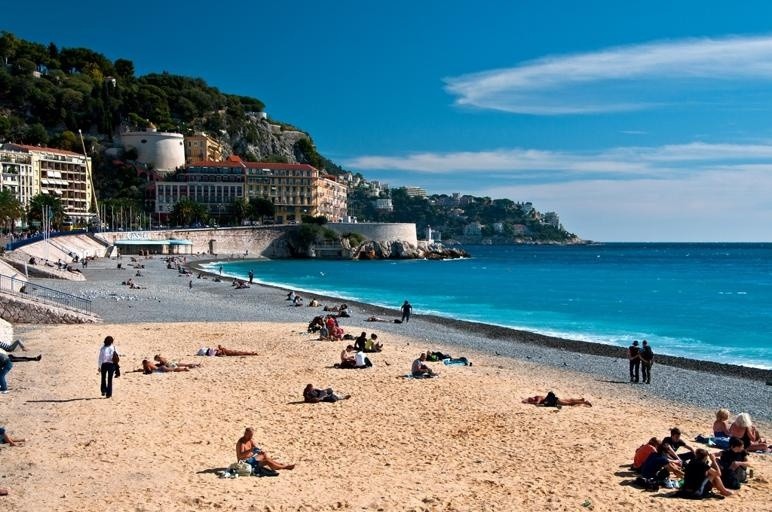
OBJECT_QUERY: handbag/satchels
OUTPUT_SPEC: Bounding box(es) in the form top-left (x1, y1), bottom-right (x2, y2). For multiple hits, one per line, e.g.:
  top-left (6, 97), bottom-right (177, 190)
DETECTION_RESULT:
top-left (230, 460), bottom-right (253, 477)
top-left (112, 345), bottom-right (119, 364)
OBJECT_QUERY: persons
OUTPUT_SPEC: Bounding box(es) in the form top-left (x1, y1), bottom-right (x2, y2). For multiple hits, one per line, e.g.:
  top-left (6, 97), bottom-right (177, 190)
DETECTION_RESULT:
top-left (0, 351), bottom-right (15, 394)
top-left (284, 289), bottom-right (383, 367)
top-left (1, 426), bottom-right (26, 446)
top-left (216, 344), bottom-right (258, 356)
top-left (154, 353), bottom-right (202, 368)
top-left (401, 300), bottom-right (412, 323)
top-left (522, 391), bottom-right (592, 407)
top-left (96, 335), bottom-right (121, 398)
top-left (236, 426), bottom-right (297, 470)
top-left (425, 347), bottom-right (450, 360)
top-left (302, 383), bottom-right (351, 402)
top-left (143, 359), bottom-right (190, 374)
top-left (632, 409), bottom-right (767, 499)
top-left (30, 248), bottom-right (254, 289)
top-left (411, 353), bottom-right (437, 378)
top-left (627, 340), bottom-right (640, 382)
top-left (638, 339), bottom-right (654, 384)
